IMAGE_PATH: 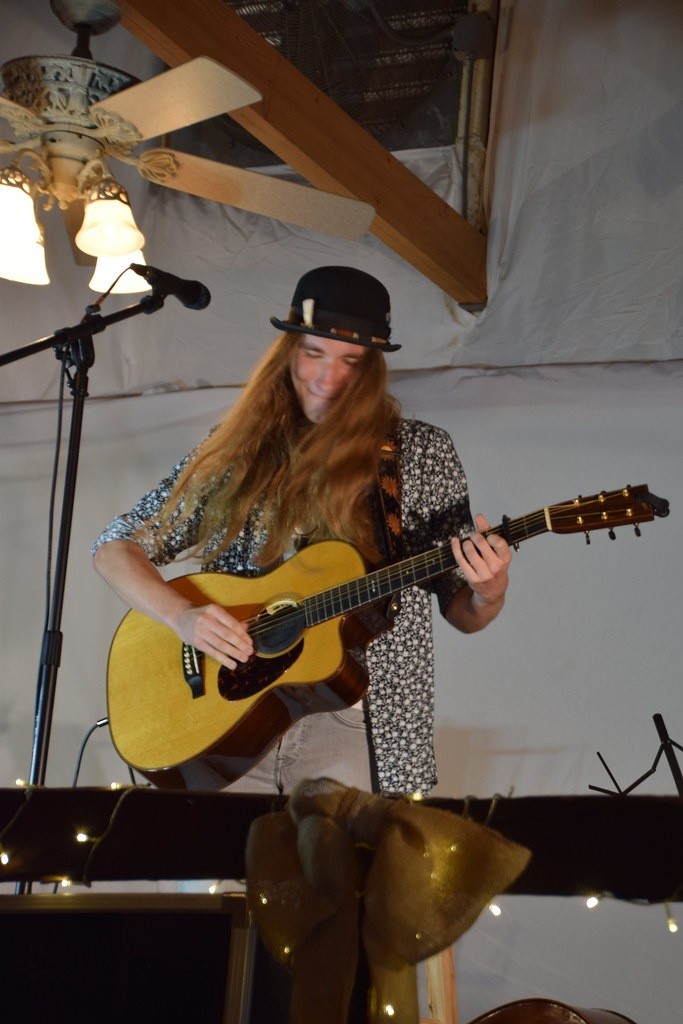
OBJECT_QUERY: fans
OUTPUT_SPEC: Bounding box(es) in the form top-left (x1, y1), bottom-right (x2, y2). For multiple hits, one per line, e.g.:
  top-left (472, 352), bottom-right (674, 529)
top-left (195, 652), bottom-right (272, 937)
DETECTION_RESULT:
top-left (0, 0), bottom-right (376, 265)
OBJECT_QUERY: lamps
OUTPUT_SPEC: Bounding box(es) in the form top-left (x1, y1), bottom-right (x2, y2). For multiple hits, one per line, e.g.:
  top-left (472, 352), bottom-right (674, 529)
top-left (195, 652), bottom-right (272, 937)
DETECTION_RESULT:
top-left (0, 147), bottom-right (154, 294)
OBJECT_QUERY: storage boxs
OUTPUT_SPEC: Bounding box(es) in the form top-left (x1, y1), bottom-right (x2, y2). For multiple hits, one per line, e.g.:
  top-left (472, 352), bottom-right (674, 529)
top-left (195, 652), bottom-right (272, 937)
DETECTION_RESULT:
top-left (0, 891), bottom-right (286, 1023)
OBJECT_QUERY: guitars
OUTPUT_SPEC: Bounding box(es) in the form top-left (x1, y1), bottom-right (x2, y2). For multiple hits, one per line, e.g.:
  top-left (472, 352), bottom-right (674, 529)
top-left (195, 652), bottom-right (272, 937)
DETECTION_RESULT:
top-left (104, 481), bottom-right (672, 793)
top-left (466, 996), bottom-right (639, 1024)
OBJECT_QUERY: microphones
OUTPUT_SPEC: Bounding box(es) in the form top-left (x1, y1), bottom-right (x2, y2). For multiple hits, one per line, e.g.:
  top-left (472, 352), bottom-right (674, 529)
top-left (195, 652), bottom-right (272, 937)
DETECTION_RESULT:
top-left (130, 263), bottom-right (212, 310)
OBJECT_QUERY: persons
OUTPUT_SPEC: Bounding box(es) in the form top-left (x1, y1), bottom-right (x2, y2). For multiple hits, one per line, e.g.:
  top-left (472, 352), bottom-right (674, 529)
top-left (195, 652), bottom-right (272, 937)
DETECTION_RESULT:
top-left (92, 265), bottom-right (513, 793)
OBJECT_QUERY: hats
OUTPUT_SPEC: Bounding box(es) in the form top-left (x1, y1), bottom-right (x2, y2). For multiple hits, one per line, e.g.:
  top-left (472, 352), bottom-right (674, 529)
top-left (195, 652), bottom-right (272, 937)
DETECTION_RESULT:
top-left (271, 265), bottom-right (402, 352)
